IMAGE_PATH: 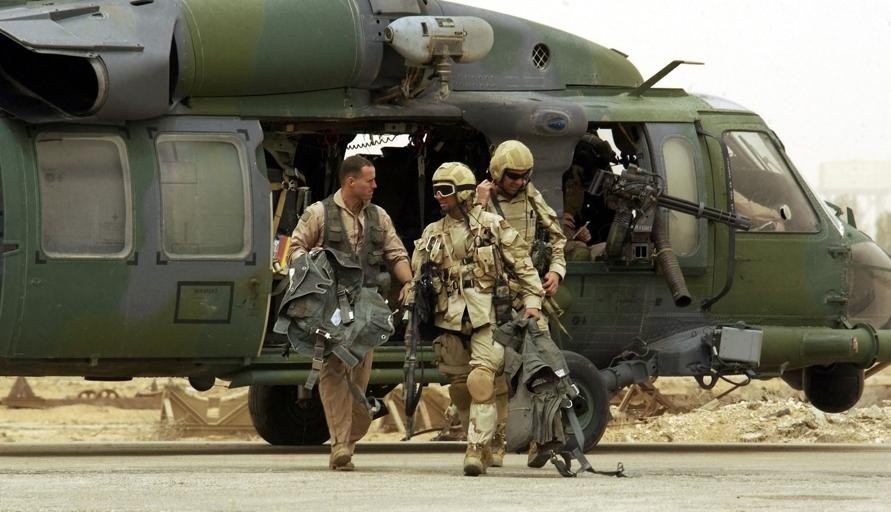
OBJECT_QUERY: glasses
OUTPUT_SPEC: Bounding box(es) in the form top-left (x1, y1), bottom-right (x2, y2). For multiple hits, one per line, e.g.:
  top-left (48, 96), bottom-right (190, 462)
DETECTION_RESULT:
top-left (505, 170), bottom-right (529, 180)
top-left (433, 183), bottom-right (477, 197)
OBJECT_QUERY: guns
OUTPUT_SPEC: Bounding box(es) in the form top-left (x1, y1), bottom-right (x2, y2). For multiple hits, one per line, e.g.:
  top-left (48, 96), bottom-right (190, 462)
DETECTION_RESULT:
top-left (401, 263), bottom-right (432, 442)
top-left (585, 163), bottom-right (750, 233)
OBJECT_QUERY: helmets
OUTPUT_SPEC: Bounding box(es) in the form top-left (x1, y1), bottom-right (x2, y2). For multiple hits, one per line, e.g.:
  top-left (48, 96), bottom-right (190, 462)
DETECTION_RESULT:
top-left (490, 139), bottom-right (533, 183)
top-left (432, 162), bottom-right (476, 203)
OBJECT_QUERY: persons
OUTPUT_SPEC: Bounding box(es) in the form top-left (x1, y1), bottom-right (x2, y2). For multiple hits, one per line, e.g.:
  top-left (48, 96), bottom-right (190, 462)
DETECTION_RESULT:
top-left (260, 138), bottom-right (307, 347)
top-left (473, 140), bottom-right (568, 467)
top-left (563, 209), bottom-right (607, 262)
top-left (403, 161), bottom-right (546, 478)
top-left (287, 154), bottom-right (414, 471)
top-left (563, 121), bottom-right (617, 243)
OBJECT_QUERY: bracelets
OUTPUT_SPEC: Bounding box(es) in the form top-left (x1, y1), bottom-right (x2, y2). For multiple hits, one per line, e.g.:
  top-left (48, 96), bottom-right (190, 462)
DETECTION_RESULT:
top-left (404, 279), bottom-right (412, 286)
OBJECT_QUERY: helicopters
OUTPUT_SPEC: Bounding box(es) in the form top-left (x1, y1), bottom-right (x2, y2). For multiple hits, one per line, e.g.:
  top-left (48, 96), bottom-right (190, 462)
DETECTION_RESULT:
top-left (0, 0), bottom-right (891, 454)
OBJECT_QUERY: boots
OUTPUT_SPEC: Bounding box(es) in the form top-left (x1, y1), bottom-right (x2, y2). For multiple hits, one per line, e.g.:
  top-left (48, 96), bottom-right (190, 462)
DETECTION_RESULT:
top-left (329, 443), bottom-right (354, 471)
top-left (527, 439), bottom-right (551, 468)
top-left (463, 432), bottom-right (505, 475)
